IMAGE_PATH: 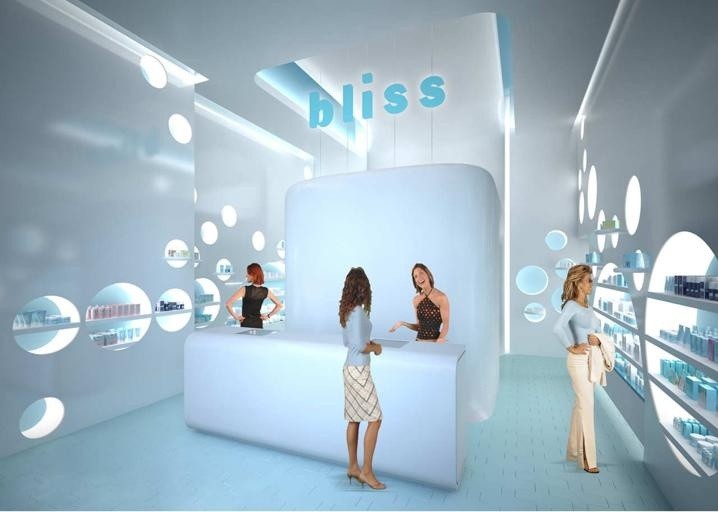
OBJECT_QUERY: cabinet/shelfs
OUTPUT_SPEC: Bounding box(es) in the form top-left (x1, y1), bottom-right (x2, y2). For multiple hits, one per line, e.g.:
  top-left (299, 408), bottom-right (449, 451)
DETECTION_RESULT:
top-left (582, 279), bottom-right (647, 405)
top-left (646, 285), bottom-right (716, 479)
top-left (87, 313), bottom-right (157, 352)
top-left (192, 301), bottom-right (221, 328)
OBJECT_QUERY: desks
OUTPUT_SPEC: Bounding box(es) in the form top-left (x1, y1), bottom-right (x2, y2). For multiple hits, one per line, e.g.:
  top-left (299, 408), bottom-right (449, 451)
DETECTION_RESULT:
top-left (179, 322), bottom-right (472, 495)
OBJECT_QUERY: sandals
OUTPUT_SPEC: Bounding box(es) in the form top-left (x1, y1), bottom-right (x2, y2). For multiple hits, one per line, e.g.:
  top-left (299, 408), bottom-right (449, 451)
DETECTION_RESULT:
top-left (585, 464), bottom-right (599, 473)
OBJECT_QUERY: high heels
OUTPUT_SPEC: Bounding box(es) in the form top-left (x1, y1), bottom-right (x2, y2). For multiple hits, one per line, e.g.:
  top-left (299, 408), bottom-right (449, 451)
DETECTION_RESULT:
top-left (347, 471), bottom-right (384, 489)
